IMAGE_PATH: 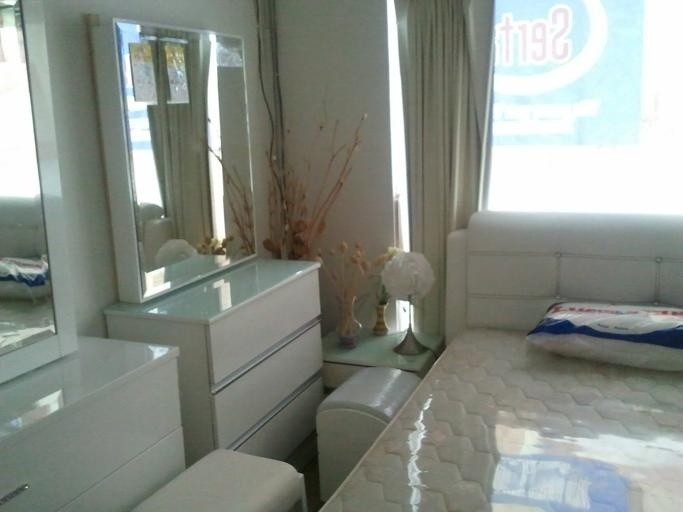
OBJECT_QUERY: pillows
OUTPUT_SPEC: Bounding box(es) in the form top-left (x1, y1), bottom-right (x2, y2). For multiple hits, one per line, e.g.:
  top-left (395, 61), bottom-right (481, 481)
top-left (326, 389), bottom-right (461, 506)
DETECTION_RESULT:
top-left (525, 300), bottom-right (683, 373)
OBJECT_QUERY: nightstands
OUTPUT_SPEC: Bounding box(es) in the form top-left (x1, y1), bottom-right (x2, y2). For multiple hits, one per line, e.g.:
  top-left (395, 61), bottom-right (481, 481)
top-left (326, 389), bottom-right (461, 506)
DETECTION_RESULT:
top-left (322, 327), bottom-right (443, 389)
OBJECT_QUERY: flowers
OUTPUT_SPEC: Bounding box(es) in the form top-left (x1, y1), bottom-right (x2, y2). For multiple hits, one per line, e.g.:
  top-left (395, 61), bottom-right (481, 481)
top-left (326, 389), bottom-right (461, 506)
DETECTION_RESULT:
top-left (316, 241), bottom-right (401, 306)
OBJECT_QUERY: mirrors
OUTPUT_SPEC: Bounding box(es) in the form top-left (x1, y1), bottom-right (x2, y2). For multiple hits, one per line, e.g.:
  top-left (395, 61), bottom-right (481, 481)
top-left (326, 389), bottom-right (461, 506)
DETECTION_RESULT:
top-left (90, 13), bottom-right (255, 305)
top-left (1, 0), bottom-right (80, 386)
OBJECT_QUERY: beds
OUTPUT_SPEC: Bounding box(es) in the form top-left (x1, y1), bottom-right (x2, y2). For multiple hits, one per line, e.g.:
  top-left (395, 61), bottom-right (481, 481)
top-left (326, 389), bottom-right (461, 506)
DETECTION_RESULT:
top-left (311, 210), bottom-right (683, 512)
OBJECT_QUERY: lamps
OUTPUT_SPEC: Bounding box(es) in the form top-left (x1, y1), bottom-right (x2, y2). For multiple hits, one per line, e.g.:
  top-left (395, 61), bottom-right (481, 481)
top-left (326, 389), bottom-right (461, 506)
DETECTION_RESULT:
top-left (380, 251), bottom-right (436, 356)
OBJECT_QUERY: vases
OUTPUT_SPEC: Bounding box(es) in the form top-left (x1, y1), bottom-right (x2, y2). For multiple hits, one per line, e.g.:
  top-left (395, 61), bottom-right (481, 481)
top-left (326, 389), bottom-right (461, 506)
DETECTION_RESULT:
top-left (335, 295), bottom-right (389, 349)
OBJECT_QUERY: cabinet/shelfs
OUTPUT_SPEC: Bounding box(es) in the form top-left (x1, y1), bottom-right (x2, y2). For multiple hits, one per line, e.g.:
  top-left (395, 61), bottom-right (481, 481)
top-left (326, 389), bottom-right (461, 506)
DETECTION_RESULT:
top-left (2, 336), bottom-right (186, 512)
top-left (100, 256), bottom-right (323, 468)
